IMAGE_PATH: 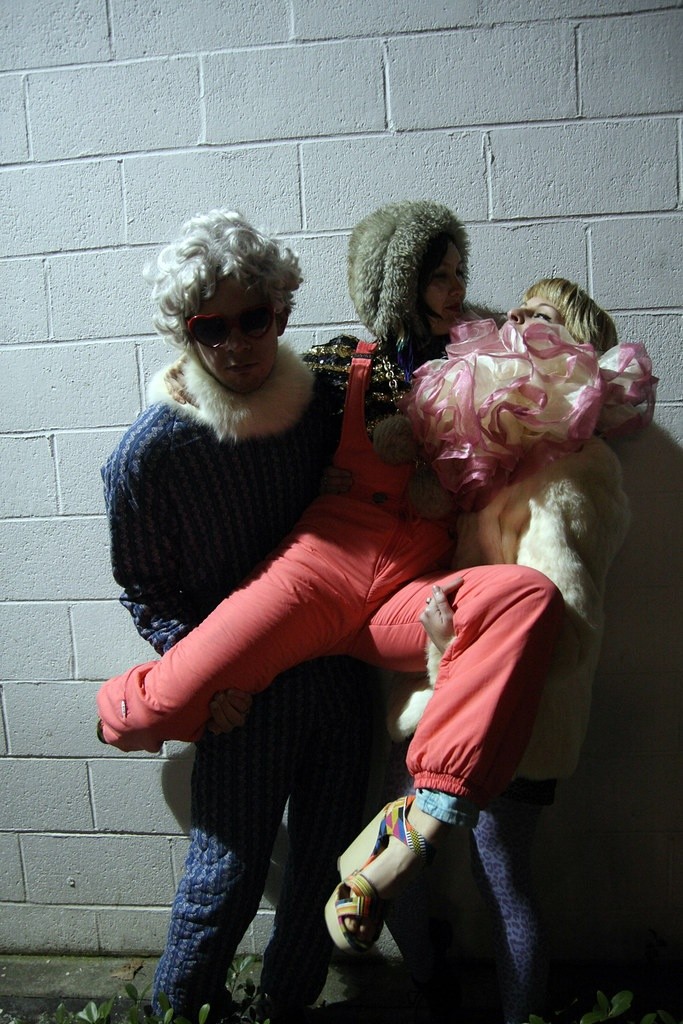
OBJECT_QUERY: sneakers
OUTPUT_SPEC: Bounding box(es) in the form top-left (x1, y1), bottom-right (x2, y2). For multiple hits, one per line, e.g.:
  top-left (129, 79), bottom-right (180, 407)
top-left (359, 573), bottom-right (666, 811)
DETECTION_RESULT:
top-left (407, 916), bottom-right (464, 1018)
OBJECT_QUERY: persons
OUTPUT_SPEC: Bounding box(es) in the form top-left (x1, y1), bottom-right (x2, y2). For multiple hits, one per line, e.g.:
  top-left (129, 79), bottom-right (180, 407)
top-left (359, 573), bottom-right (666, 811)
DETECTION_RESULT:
top-left (98, 201), bottom-right (560, 955)
top-left (100, 210), bottom-right (372, 1024)
top-left (420, 278), bottom-right (632, 1024)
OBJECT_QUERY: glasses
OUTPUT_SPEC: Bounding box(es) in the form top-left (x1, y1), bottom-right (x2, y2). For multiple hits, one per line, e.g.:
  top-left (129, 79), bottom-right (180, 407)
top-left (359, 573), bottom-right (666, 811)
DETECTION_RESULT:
top-left (185, 301), bottom-right (276, 347)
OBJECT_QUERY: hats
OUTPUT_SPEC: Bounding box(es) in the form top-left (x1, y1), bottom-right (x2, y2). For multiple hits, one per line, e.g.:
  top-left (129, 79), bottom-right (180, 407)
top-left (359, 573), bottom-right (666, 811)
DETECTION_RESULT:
top-left (349, 200), bottom-right (472, 343)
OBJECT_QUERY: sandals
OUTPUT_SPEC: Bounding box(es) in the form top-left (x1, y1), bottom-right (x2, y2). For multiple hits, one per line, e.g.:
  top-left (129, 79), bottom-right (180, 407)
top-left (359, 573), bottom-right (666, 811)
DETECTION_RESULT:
top-left (324, 794), bottom-right (434, 950)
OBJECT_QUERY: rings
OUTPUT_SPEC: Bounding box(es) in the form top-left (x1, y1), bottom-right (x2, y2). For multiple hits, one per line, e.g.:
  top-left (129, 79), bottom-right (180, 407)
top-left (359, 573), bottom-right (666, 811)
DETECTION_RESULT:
top-left (423, 596), bottom-right (433, 603)
